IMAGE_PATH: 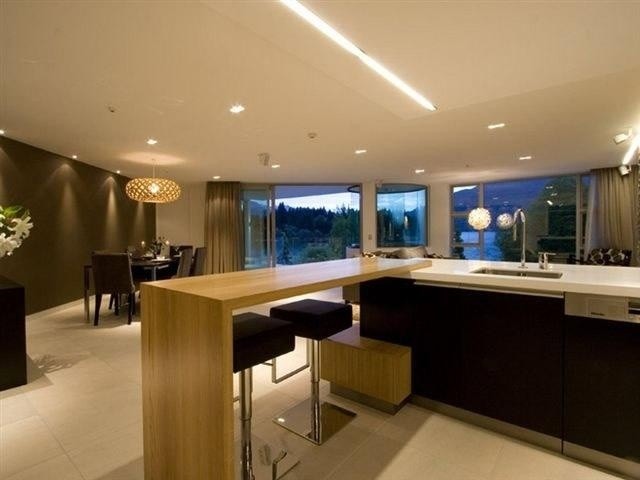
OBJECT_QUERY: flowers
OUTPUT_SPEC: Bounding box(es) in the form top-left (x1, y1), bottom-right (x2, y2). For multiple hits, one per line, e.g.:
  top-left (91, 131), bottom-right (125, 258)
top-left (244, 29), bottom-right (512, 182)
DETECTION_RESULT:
top-left (0, 205), bottom-right (34, 258)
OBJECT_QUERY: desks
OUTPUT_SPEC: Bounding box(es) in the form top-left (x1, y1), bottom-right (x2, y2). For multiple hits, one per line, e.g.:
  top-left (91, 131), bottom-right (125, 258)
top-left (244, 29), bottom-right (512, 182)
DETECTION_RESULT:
top-left (84, 255), bottom-right (195, 323)
top-left (0, 275), bottom-right (27, 391)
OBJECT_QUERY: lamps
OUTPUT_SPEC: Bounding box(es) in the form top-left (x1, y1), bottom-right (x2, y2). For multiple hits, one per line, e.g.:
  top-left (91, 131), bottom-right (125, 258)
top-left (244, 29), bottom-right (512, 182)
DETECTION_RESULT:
top-left (468, 207), bottom-right (515, 231)
top-left (126, 159), bottom-right (181, 203)
top-left (612, 127), bottom-right (640, 176)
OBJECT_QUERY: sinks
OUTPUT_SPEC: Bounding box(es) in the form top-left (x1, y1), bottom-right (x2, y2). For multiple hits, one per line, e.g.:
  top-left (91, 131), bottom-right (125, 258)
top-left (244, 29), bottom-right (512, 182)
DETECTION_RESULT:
top-left (469, 267), bottom-right (562, 279)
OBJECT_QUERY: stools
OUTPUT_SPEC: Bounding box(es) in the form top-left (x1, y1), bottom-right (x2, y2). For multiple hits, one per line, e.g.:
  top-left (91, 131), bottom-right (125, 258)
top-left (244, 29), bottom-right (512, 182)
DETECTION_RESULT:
top-left (270, 299), bottom-right (352, 446)
top-left (233, 312), bottom-right (300, 480)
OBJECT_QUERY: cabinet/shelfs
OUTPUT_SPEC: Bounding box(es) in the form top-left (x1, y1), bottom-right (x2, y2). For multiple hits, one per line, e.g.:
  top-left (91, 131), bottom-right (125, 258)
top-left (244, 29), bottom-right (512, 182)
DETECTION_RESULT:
top-left (413, 280), bottom-right (564, 454)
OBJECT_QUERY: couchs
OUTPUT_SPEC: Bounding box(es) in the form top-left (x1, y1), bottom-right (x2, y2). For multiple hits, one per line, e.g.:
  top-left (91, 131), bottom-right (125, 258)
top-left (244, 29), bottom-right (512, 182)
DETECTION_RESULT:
top-left (583, 248), bottom-right (631, 266)
top-left (373, 246), bottom-right (445, 258)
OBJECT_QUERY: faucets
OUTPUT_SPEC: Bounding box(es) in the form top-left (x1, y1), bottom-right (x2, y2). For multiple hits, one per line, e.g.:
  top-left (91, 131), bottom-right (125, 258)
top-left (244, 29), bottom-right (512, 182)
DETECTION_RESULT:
top-left (513, 209), bottom-right (526, 265)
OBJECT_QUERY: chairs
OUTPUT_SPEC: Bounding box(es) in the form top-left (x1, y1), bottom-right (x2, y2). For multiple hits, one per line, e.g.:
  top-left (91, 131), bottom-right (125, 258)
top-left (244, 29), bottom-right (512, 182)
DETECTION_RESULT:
top-left (92, 246), bottom-right (208, 326)
